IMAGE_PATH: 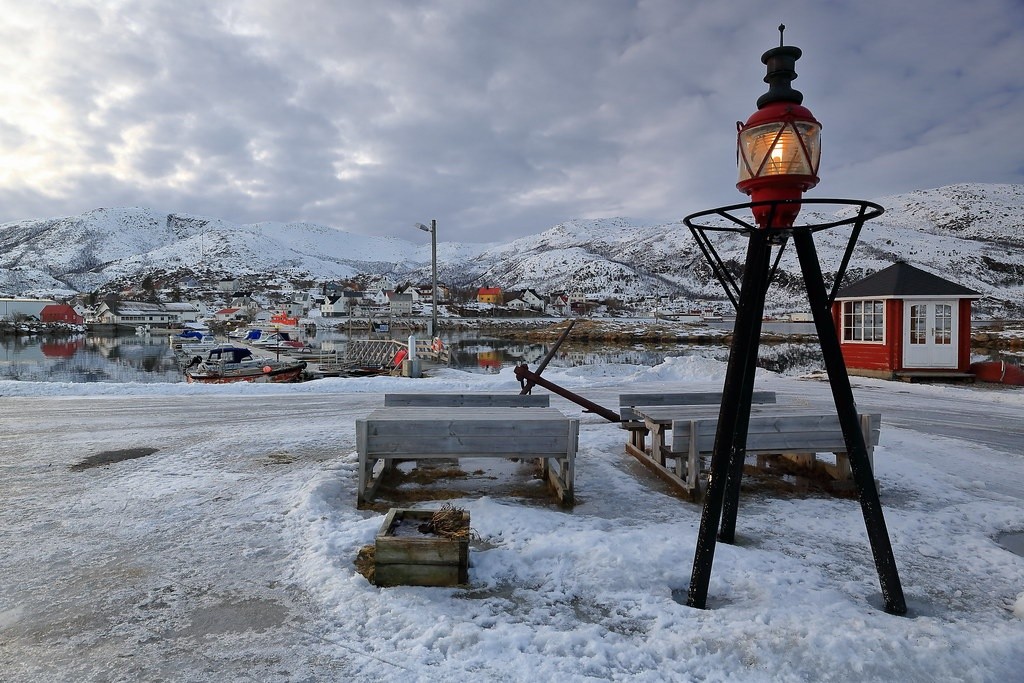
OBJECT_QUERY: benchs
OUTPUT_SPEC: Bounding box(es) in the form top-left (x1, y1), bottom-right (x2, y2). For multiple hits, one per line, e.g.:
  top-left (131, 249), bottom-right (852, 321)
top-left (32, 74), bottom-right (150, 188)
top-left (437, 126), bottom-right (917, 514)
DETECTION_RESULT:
top-left (620, 391), bottom-right (881, 493)
top-left (355, 394), bottom-right (579, 506)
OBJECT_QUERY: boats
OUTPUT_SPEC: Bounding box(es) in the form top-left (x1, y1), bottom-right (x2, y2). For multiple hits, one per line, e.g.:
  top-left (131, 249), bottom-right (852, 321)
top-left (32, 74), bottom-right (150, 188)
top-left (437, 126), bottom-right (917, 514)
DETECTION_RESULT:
top-left (168, 326), bottom-right (347, 384)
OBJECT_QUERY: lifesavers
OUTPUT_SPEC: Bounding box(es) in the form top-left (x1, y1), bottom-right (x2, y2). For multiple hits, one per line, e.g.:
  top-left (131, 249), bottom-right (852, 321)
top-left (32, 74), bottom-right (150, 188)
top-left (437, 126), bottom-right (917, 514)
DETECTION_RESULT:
top-left (432, 337), bottom-right (440, 355)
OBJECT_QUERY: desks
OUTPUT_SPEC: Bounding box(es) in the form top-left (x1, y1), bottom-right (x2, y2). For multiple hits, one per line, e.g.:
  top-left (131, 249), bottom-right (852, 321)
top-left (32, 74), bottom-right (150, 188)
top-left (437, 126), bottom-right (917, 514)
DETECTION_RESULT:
top-left (368, 407), bottom-right (567, 472)
top-left (632, 404), bottom-right (836, 468)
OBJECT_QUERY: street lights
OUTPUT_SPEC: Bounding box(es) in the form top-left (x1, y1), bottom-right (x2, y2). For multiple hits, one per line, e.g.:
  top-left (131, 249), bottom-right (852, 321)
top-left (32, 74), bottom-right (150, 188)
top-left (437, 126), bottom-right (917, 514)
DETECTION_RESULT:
top-left (415, 219), bottom-right (437, 361)
